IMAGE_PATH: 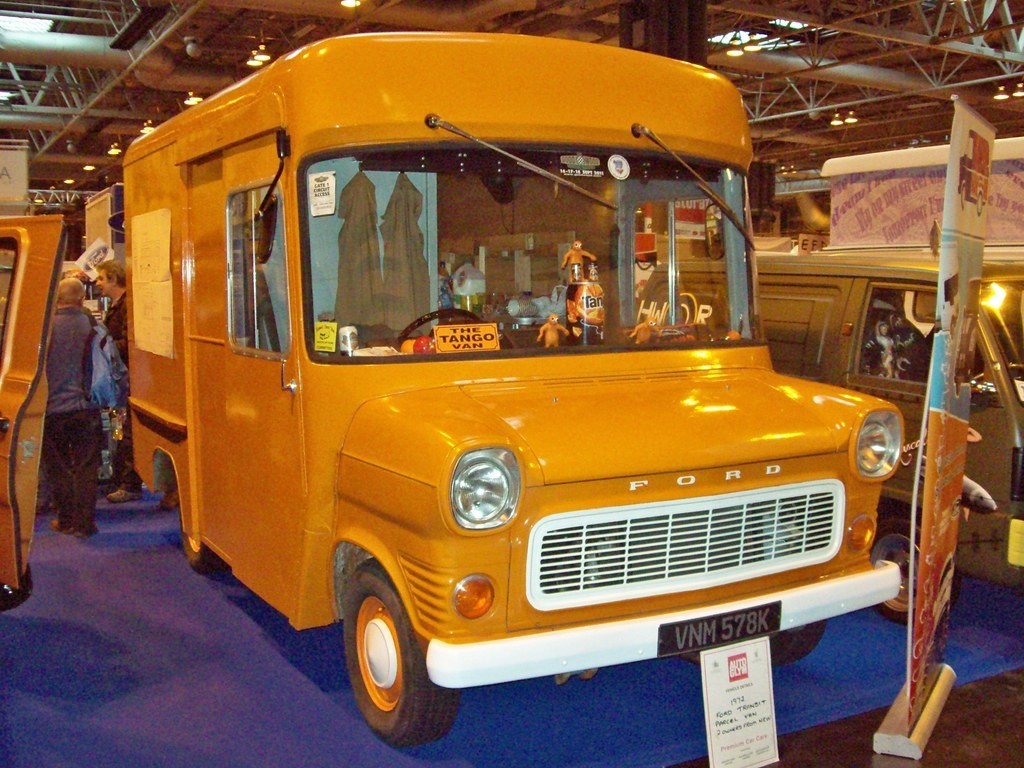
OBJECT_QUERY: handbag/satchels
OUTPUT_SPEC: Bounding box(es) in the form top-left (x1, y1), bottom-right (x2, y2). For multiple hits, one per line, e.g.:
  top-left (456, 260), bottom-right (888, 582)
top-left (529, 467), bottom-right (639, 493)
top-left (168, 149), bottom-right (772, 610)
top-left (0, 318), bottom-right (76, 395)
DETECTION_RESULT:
top-left (82, 313), bottom-right (128, 409)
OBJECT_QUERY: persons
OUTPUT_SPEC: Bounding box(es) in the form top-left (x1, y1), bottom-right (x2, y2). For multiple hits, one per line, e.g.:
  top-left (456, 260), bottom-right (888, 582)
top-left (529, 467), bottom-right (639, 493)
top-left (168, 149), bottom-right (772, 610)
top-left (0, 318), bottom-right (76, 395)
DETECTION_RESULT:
top-left (34, 259), bottom-right (143, 537)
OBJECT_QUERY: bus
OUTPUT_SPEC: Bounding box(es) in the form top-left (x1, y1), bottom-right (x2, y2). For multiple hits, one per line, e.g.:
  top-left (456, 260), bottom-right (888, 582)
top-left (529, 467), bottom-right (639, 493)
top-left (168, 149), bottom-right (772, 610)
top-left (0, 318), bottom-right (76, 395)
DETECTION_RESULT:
top-left (117, 30), bottom-right (910, 752)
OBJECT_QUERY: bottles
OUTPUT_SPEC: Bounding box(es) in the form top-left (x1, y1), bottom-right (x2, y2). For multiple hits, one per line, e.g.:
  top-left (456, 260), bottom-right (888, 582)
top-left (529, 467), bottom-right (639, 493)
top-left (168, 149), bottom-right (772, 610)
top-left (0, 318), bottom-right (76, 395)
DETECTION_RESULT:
top-left (587, 261), bottom-right (599, 282)
top-left (109, 406), bottom-right (123, 440)
top-left (571, 262), bottom-right (582, 282)
top-left (453, 259), bottom-right (486, 320)
top-left (438, 261), bottom-right (453, 309)
top-left (519, 291), bottom-right (537, 316)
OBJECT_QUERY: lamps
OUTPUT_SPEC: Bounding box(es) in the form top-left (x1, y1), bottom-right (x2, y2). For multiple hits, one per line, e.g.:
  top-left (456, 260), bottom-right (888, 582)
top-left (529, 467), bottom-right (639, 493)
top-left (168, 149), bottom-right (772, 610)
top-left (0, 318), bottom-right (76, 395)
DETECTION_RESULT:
top-left (1012, 80), bottom-right (1024, 97)
top-left (845, 109), bottom-right (858, 124)
top-left (830, 112), bottom-right (843, 126)
top-left (33, 0), bottom-right (362, 202)
top-left (993, 83), bottom-right (1009, 100)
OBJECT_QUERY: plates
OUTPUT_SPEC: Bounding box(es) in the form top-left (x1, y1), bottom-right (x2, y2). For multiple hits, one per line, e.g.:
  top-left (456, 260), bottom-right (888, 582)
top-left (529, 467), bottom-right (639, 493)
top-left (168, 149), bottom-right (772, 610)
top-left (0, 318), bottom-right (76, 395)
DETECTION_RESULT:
top-left (517, 318), bottom-right (537, 326)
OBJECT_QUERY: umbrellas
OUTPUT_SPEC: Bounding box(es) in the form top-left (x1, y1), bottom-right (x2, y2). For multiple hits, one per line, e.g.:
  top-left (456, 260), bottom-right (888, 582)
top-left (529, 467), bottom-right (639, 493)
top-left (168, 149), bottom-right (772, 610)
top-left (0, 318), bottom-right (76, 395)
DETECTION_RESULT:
top-left (379, 170), bottom-right (431, 337)
top-left (334, 164), bottom-right (383, 328)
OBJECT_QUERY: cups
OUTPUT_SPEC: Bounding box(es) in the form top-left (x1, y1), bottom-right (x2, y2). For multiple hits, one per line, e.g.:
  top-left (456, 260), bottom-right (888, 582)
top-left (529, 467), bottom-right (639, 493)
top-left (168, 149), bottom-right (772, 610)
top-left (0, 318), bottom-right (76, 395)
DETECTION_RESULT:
top-left (461, 295), bottom-right (473, 313)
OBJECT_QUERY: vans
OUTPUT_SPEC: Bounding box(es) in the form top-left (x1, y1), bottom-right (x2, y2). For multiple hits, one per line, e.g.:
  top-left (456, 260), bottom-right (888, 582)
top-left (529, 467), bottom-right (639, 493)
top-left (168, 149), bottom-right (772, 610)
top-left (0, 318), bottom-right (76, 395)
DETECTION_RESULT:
top-left (637, 245), bottom-right (1023, 624)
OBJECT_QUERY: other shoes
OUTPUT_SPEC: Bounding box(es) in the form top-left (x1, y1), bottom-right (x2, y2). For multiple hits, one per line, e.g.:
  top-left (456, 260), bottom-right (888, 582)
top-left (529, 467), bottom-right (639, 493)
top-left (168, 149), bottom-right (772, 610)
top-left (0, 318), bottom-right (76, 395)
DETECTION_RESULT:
top-left (107, 490), bottom-right (142, 502)
top-left (74, 523), bottom-right (97, 538)
top-left (36, 505), bottom-right (48, 514)
top-left (50, 520), bottom-right (73, 534)
top-left (160, 495), bottom-right (177, 510)
